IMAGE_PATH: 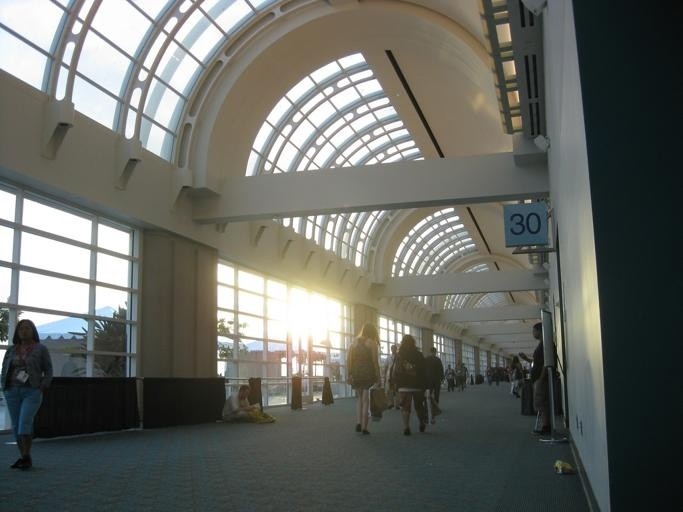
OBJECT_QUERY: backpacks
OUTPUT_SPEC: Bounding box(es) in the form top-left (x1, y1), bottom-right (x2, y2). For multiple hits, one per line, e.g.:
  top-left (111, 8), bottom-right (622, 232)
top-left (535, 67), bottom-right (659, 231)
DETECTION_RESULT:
top-left (398, 360), bottom-right (417, 385)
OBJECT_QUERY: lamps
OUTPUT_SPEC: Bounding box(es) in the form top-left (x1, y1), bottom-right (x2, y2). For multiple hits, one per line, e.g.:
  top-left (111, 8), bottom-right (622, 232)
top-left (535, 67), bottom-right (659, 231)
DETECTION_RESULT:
top-left (534, 135), bottom-right (551, 151)
top-left (521, 0), bottom-right (546, 16)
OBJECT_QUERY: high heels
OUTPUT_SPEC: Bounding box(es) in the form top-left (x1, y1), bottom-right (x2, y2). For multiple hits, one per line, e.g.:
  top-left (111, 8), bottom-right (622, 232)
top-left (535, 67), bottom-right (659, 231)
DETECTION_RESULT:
top-left (419, 424), bottom-right (425, 432)
top-left (404, 428), bottom-right (410, 435)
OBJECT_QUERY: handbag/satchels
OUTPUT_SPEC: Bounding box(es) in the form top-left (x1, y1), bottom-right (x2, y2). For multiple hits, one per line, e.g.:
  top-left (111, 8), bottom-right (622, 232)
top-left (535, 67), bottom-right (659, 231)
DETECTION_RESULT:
top-left (428, 395), bottom-right (442, 424)
top-left (370, 388), bottom-right (387, 417)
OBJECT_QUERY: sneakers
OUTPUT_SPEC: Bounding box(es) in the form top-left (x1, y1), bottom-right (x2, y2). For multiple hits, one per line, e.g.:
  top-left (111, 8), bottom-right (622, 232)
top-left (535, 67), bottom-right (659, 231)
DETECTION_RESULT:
top-left (529, 428), bottom-right (549, 438)
top-left (356, 424), bottom-right (369, 434)
top-left (11, 458), bottom-right (32, 469)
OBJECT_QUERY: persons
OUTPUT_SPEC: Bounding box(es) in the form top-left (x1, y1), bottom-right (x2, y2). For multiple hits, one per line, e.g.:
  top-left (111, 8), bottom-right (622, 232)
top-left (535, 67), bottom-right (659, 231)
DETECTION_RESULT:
top-left (385, 335), bottom-right (433, 435)
top-left (445, 362), bottom-right (468, 392)
top-left (486, 352), bottom-right (531, 398)
top-left (531, 323), bottom-right (557, 435)
top-left (347, 323), bottom-right (380, 435)
top-left (222, 385), bottom-right (257, 422)
top-left (0, 319), bottom-right (54, 470)
top-left (426, 347), bottom-right (444, 406)
